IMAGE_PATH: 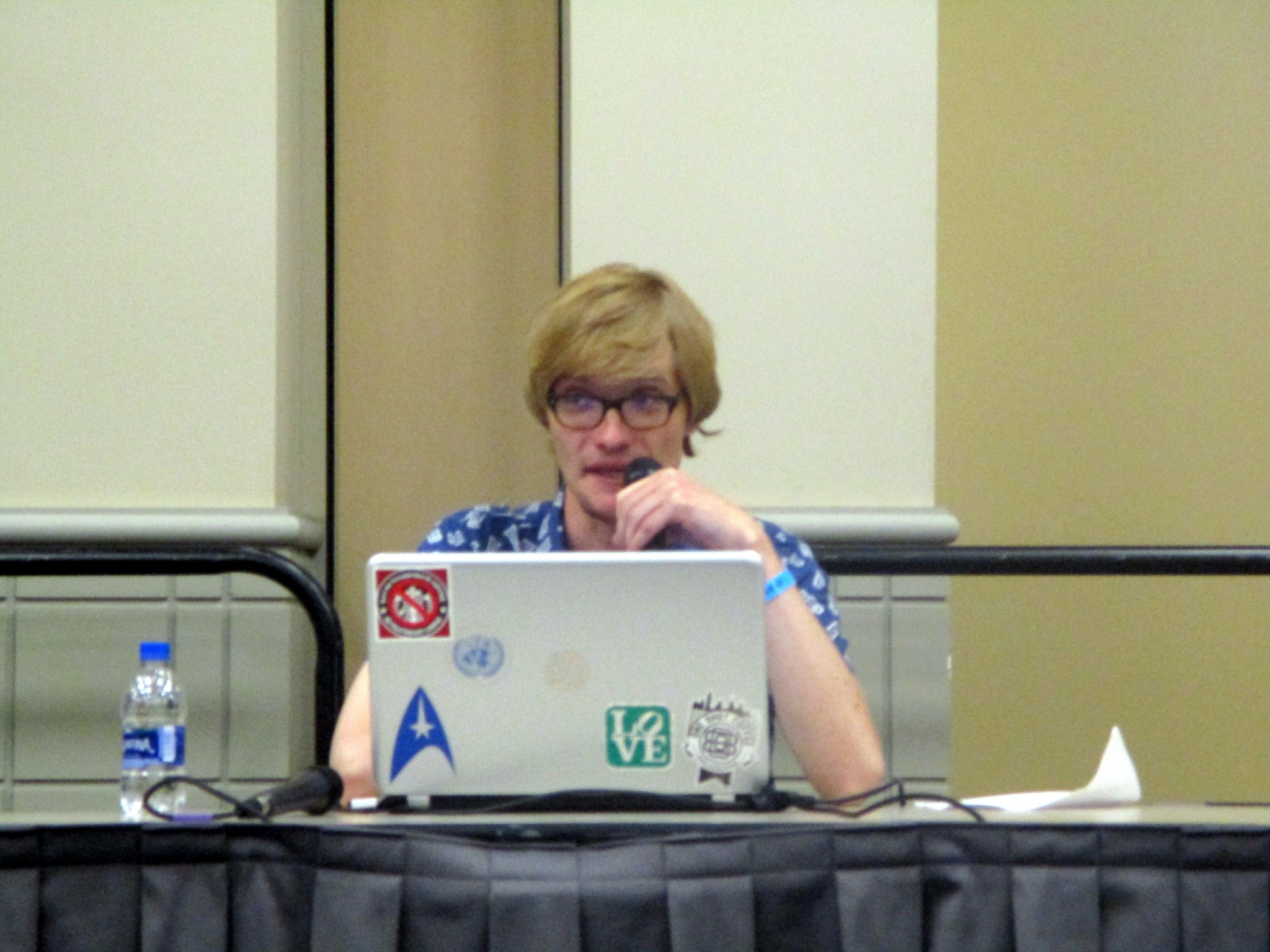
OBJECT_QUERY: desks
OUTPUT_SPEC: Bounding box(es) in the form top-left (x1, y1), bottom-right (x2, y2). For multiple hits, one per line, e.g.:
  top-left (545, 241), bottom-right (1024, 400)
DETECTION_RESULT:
top-left (0, 807), bottom-right (1270, 952)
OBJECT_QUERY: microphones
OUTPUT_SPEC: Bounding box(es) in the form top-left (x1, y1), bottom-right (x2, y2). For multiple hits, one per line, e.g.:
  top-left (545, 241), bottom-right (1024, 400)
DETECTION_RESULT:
top-left (236, 764), bottom-right (344, 816)
top-left (624, 457), bottom-right (668, 551)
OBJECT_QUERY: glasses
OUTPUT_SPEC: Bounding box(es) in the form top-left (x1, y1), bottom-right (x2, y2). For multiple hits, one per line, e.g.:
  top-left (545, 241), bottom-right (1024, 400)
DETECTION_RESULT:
top-left (545, 385), bottom-right (683, 432)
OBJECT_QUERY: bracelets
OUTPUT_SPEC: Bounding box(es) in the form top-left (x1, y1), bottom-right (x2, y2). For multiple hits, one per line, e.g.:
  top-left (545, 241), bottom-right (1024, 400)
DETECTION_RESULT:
top-left (765, 567), bottom-right (798, 604)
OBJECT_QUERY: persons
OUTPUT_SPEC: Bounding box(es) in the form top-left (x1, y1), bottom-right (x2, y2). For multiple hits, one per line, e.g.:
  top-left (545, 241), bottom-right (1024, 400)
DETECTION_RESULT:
top-left (329, 263), bottom-right (884, 804)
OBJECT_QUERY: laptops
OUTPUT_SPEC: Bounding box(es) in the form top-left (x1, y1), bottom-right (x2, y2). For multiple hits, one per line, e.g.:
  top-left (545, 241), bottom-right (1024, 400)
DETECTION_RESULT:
top-left (360, 552), bottom-right (771, 810)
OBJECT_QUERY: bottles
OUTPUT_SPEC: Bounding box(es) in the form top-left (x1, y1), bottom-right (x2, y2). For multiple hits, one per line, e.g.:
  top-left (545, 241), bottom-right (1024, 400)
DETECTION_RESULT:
top-left (120, 641), bottom-right (186, 820)
